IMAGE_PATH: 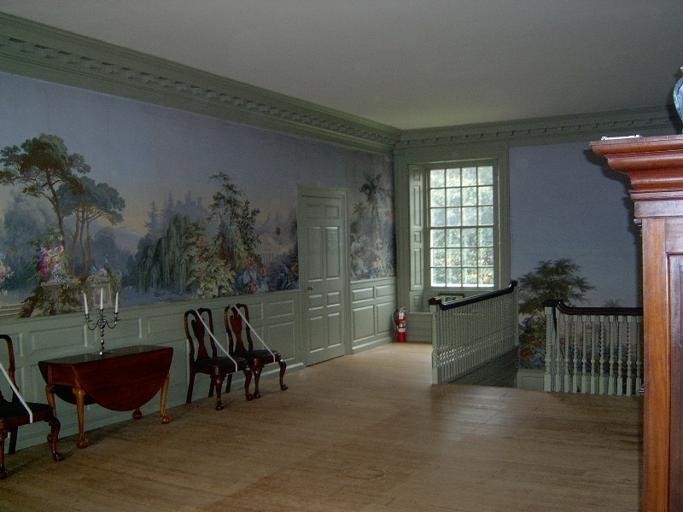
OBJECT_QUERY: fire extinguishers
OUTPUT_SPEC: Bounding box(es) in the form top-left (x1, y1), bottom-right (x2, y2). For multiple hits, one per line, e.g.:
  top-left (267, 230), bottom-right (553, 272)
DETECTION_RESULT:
top-left (393, 307), bottom-right (407, 342)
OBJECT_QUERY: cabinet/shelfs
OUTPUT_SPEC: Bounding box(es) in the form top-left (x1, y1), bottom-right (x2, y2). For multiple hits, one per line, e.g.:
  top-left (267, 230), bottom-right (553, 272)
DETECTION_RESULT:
top-left (588, 133), bottom-right (682, 511)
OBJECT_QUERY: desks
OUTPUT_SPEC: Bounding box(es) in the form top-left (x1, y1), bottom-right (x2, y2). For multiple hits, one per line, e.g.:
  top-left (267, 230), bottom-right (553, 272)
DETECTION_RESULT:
top-left (37, 344), bottom-right (174, 448)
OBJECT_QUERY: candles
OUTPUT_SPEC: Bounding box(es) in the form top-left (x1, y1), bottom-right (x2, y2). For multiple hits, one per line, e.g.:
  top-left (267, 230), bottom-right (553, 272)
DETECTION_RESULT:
top-left (113, 291), bottom-right (118, 313)
top-left (82, 292), bottom-right (88, 314)
top-left (99, 287), bottom-right (103, 310)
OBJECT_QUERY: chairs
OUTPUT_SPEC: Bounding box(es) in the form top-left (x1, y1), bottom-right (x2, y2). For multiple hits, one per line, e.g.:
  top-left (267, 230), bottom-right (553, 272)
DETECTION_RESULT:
top-left (183, 308), bottom-right (252, 411)
top-left (0, 334), bottom-right (65, 478)
top-left (224, 304), bottom-right (288, 400)
